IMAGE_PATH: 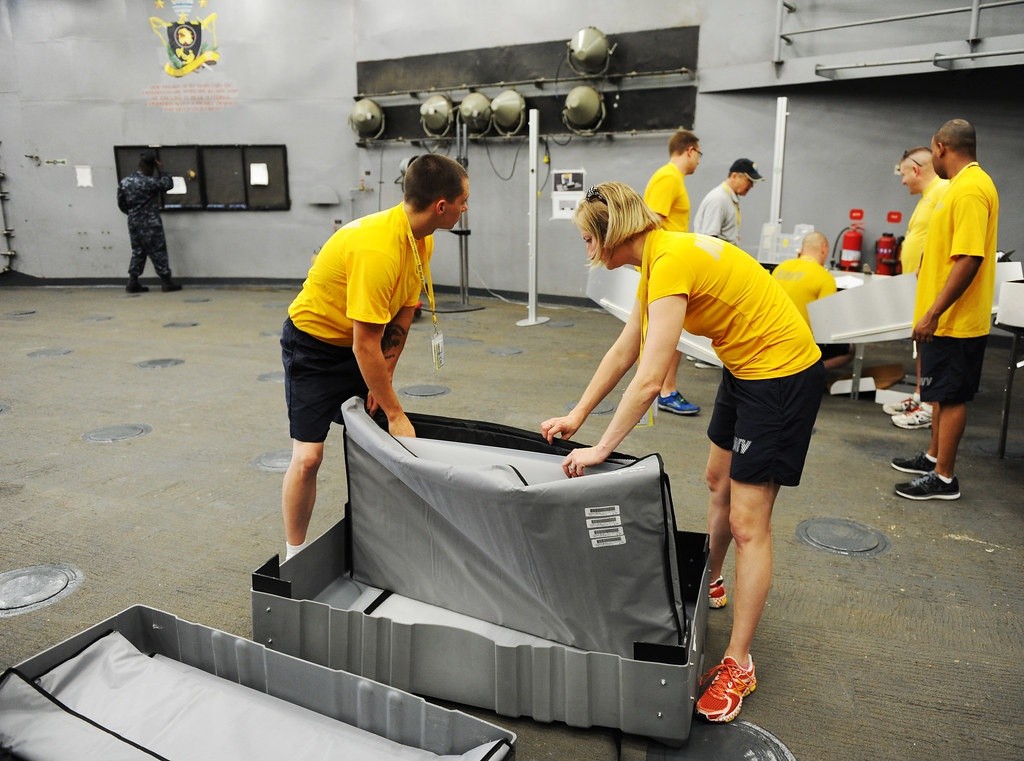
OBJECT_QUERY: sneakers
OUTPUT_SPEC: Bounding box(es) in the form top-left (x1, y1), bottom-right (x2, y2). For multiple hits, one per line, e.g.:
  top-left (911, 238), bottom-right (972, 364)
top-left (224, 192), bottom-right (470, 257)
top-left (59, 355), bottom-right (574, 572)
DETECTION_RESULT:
top-left (708, 575), bottom-right (727, 608)
top-left (890, 406), bottom-right (933, 430)
top-left (695, 653), bottom-right (757, 723)
top-left (891, 451), bottom-right (937, 475)
top-left (657, 392), bottom-right (701, 415)
top-left (895, 471), bottom-right (961, 500)
top-left (882, 397), bottom-right (919, 415)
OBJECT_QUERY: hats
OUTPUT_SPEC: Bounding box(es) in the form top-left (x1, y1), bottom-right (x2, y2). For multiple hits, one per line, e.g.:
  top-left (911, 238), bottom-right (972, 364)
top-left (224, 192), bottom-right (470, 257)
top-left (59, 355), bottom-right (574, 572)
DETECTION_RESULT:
top-left (140, 149), bottom-right (160, 167)
top-left (729, 158), bottom-right (766, 182)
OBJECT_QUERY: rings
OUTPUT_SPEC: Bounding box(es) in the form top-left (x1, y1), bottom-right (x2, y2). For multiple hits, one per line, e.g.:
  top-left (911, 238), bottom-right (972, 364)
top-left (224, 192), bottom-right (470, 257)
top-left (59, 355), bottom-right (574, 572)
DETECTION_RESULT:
top-left (549, 423), bottom-right (552, 427)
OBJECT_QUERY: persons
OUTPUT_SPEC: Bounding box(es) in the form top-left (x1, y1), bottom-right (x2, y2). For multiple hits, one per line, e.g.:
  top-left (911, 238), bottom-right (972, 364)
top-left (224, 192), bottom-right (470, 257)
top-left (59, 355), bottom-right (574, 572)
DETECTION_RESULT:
top-left (883, 145), bottom-right (950, 430)
top-left (686, 157), bottom-right (764, 369)
top-left (539, 181), bottom-right (824, 723)
top-left (632, 130), bottom-right (704, 415)
top-left (771, 231), bottom-right (855, 369)
top-left (117, 151), bottom-right (182, 292)
top-left (280, 152), bottom-right (470, 559)
top-left (891, 119), bottom-right (998, 501)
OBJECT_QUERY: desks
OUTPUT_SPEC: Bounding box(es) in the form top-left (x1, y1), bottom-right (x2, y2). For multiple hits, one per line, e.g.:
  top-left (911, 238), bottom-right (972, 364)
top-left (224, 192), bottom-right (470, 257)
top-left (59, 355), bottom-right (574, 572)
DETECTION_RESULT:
top-left (757, 260), bottom-right (1024, 461)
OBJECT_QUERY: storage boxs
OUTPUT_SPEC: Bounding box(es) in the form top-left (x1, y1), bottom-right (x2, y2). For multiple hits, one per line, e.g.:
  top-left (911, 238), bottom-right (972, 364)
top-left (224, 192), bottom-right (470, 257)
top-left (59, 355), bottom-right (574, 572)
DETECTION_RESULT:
top-left (830, 363), bottom-right (921, 405)
top-left (0, 503), bottom-right (711, 760)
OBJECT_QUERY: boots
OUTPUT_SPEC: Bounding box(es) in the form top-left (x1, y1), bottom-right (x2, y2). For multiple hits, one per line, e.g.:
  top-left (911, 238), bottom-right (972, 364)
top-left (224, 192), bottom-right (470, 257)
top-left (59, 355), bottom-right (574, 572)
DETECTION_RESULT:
top-left (125, 273), bottom-right (150, 293)
top-left (160, 272), bottom-right (182, 292)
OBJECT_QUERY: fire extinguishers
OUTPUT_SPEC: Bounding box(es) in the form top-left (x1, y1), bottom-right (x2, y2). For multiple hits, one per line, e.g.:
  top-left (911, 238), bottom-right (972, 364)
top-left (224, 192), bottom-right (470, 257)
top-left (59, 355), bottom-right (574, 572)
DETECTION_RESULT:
top-left (877, 234), bottom-right (904, 275)
top-left (839, 222), bottom-right (865, 273)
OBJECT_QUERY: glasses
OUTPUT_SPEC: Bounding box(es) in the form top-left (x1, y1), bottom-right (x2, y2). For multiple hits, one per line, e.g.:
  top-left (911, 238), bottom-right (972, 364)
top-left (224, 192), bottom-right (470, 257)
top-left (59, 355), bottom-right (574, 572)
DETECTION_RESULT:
top-left (686, 147), bottom-right (703, 157)
top-left (586, 184), bottom-right (607, 207)
top-left (903, 150), bottom-right (922, 168)
